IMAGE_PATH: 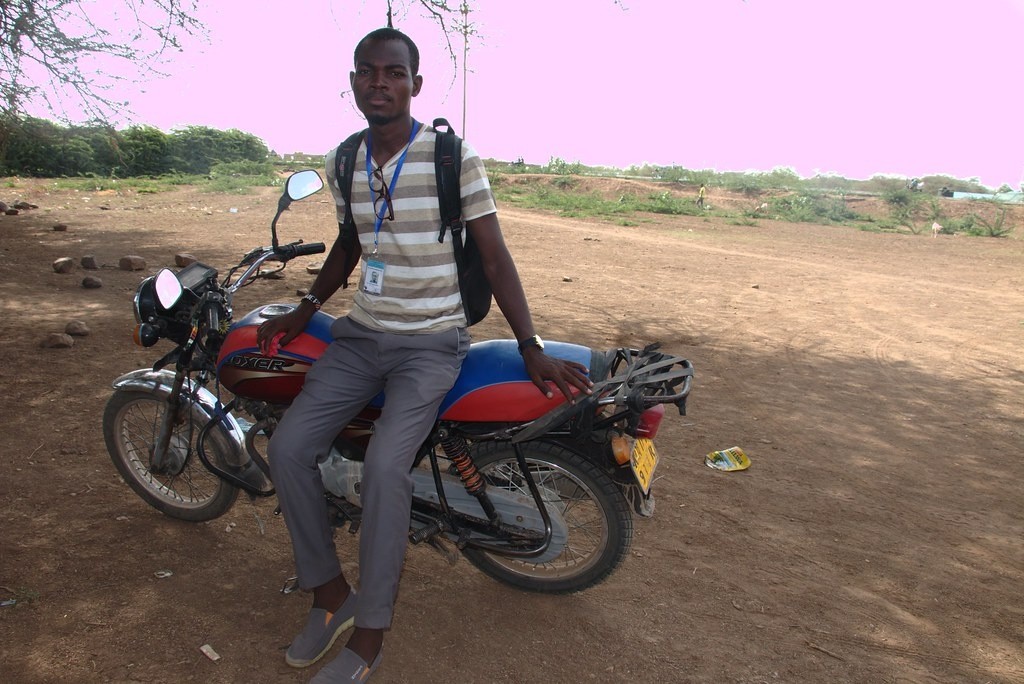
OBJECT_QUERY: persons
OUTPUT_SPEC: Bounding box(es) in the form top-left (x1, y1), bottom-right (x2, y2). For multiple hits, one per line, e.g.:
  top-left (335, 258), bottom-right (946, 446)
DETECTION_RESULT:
top-left (255, 27), bottom-right (594, 683)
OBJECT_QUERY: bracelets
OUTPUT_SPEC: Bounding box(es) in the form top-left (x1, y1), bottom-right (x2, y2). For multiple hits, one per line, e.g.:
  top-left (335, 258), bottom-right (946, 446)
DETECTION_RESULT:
top-left (300, 293), bottom-right (322, 312)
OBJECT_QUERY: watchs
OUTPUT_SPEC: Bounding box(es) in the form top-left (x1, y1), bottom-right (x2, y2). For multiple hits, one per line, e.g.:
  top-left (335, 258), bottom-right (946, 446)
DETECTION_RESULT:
top-left (516, 333), bottom-right (545, 356)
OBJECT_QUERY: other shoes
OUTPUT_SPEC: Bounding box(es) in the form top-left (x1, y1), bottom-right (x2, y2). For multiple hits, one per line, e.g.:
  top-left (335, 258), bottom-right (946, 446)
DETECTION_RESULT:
top-left (310, 638), bottom-right (383, 684)
top-left (286, 587), bottom-right (358, 667)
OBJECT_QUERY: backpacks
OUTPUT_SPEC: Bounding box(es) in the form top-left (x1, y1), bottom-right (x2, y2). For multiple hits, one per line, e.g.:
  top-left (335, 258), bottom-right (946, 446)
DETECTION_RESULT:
top-left (337, 118), bottom-right (492, 326)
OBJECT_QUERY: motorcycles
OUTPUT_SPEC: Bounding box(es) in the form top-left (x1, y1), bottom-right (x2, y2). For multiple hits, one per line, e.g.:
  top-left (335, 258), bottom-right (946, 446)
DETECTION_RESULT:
top-left (101, 169), bottom-right (698, 597)
